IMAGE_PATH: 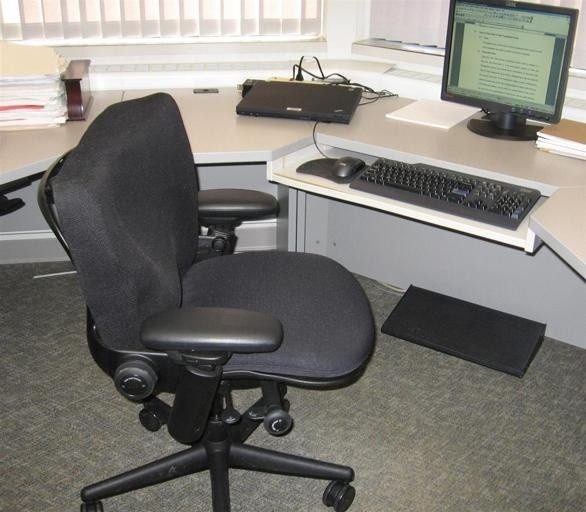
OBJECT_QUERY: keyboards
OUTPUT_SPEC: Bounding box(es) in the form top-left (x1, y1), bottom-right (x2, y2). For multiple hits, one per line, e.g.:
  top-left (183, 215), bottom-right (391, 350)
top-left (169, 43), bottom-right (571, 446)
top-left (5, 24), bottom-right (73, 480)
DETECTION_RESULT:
top-left (349, 158), bottom-right (541, 231)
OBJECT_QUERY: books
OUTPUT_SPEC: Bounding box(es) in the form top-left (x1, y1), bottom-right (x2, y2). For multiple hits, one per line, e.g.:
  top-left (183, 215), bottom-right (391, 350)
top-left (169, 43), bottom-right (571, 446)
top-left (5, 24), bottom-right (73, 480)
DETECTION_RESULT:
top-left (535, 118), bottom-right (586, 160)
top-left (385, 99), bottom-right (481, 129)
top-left (0, 49), bottom-right (69, 129)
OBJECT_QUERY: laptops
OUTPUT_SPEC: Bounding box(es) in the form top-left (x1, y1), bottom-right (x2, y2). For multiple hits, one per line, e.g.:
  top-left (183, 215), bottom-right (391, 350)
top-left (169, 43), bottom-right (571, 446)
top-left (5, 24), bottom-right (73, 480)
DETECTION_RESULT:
top-left (235, 79), bottom-right (364, 124)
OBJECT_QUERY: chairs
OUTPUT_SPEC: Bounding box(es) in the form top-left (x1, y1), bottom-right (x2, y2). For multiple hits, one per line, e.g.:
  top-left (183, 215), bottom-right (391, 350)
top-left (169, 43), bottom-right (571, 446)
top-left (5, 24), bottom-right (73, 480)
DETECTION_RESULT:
top-left (37, 93), bottom-right (376, 512)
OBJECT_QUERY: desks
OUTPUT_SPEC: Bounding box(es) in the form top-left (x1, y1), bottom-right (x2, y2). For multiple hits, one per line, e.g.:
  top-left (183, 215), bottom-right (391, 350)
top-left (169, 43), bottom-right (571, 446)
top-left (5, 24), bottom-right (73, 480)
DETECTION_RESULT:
top-left (267, 96), bottom-right (585, 353)
top-left (1, 89), bottom-right (383, 266)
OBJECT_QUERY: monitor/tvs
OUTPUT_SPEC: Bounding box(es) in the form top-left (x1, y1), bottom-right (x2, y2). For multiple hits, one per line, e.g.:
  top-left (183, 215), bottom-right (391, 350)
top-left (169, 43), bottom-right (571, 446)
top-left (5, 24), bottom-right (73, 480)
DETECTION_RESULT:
top-left (439, 0), bottom-right (579, 141)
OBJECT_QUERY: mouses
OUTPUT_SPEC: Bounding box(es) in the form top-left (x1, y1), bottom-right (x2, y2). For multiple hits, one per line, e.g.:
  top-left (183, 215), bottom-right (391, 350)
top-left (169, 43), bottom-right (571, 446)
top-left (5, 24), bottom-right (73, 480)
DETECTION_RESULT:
top-left (331, 156), bottom-right (365, 177)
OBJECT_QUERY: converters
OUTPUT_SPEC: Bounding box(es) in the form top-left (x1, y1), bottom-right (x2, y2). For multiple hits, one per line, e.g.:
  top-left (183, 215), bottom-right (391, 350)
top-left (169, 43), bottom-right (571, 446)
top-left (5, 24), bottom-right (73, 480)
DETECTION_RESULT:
top-left (241, 79), bottom-right (254, 97)
